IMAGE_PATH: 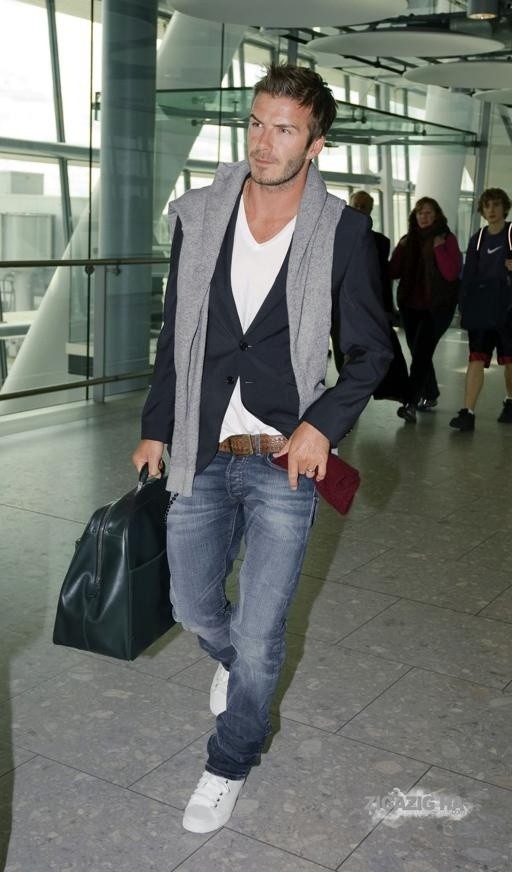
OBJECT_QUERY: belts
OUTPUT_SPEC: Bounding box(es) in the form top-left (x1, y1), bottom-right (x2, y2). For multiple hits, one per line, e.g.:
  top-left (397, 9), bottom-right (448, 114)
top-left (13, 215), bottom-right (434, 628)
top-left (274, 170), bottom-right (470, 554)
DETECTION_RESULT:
top-left (218, 433), bottom-right (289, 454)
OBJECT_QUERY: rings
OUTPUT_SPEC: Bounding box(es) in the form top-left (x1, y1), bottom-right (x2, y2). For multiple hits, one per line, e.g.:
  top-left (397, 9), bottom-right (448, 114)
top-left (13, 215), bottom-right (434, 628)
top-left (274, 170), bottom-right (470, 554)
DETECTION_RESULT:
top-left (306, 469), bottom-right (316, 473)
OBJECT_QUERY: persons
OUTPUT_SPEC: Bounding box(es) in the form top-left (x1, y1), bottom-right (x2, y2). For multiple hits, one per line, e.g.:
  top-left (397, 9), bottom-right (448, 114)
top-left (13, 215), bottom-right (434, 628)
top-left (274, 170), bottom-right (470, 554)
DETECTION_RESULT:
top-left (388, 197), bottom-right (462, 423)
top-left (131, 65), bottom-right (394, 834)
top-left (448, 188), bottom-right (512, 431)
top-left (335, 191), bottom-right (398, 374)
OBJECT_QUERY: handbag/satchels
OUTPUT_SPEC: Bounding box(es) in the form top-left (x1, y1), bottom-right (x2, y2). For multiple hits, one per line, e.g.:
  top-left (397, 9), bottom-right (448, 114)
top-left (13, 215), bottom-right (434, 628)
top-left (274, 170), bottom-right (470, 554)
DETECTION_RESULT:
top-left (51, 462), bottom-right (174, 661)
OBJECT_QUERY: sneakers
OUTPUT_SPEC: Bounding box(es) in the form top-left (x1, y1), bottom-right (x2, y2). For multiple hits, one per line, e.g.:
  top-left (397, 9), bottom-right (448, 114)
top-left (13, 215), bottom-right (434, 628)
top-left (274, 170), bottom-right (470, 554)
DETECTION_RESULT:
top-left (449, 408), bottom-right (475, 431)
top-left (183, 767), bottom-right (244, 834)
top-left (496, 398), bottom-right (512, 423)
top-left (208, 661), bottom-right (230, 715)
top-left (396, 402), bottom-right (417, 423)
top-left (416, 398), bottom-right (438, 410)
